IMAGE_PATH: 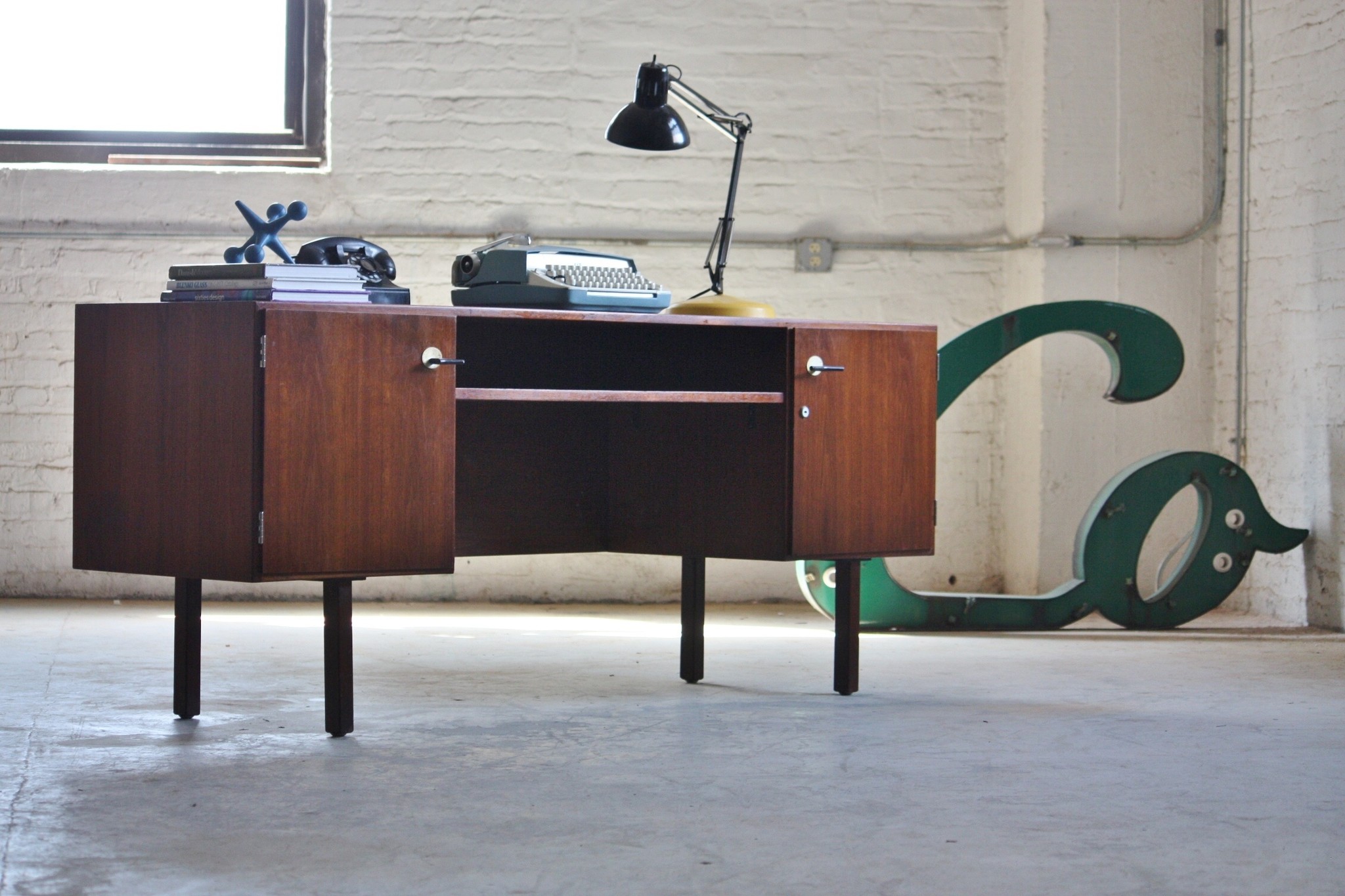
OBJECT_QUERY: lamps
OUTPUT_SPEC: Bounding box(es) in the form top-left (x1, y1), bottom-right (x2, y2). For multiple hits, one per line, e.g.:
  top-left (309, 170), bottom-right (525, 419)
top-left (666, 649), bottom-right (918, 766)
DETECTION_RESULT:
top-left (604, 52), bottom-right (780, 318)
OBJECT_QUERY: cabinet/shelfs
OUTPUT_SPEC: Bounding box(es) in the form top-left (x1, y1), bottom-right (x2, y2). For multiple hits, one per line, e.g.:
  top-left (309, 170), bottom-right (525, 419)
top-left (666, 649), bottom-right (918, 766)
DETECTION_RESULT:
top-left (75, 302), bottom-right (939, 741)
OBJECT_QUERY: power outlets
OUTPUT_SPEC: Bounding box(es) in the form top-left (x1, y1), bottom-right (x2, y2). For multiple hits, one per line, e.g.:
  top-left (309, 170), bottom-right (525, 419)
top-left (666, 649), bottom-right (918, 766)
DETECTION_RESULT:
top-left (794, 236), bottom-right (832, 271)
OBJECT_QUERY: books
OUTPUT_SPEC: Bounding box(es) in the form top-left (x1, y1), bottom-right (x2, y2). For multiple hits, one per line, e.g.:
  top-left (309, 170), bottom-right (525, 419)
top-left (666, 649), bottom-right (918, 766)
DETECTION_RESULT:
top-left (161, 263), bottom-right (373, 305)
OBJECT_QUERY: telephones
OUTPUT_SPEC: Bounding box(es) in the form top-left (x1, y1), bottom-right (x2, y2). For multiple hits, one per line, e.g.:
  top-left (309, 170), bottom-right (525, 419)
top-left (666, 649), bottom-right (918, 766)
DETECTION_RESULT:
top-left (295, 236), bottom-right (411, 305)
